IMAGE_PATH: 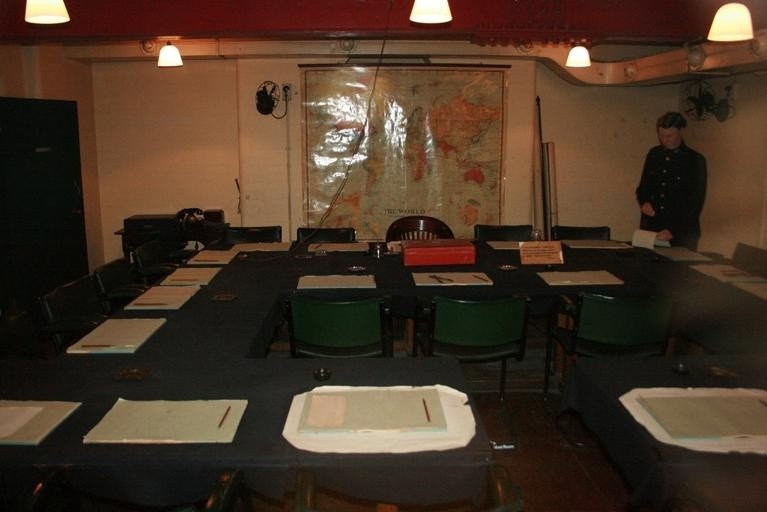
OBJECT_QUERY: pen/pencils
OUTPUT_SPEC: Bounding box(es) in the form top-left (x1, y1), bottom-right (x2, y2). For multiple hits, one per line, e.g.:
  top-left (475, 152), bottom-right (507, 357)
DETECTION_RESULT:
top-left (316, 243), bottom-right (322, 249)
top-left (172, 278), bottom-right (197, 281)
top-left (134, 302), bottom-right (168, 305)
top-left (430, 275), bottom-right (453, 281)
top-left (82, 344), bottom-right (116, 347)
top-left (218, 406), bottom-right (231, 427)
top-left (433, 274), bottom-right (443, 284)
top-left (473, 274), bottom-right (488, 282)
top-left (195, 259), bottom-right (218, 262)
top-left (422, 397), bottom-right (431, 423)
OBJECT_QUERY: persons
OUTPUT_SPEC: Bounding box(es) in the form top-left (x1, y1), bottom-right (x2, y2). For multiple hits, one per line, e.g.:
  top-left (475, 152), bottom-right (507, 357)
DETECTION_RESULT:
top-left (635, 110), bottom-right (707, 252)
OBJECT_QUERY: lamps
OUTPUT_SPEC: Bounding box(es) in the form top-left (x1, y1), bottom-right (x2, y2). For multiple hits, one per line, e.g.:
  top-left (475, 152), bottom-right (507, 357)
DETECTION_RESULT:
top-left (24, 0), bottom-right (70, 25)
top-left (565, 46), bottom-right (591, 68)
top-left (707, 3), bottom-right (754, 42)
top-left (409, 0), bottom-right (453, 24)
top-left (157, 40), bottom-right (184, 67)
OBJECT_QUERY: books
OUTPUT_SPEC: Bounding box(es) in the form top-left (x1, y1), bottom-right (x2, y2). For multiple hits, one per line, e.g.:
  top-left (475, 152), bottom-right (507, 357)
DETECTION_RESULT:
top-left (0, 399), bottom-right (83, 447)
top-left (387, 241), bottom-right (403, 252)
top-left (536, 270), bottom-right (624, 287)
top-left (185, 250), bottom-right (239, 264)
top-left (123, 285), bottom-right (200, 311)
top-left (730, 283), bottom-right (767, 300)
top-left (562, 240), bottom-right (632, 249)
top-left (654, 245), bottom-right (713, 261)
top-left (297, 274), bottom-right (377, 289)
top-left (486, 241), bottom-right (521, 250)
top-left (634, 394), bottom-right (767, 439)
top-left (308, 243), bottom-right (371, 252)
top-left (295, 390), bottom-right (447, 432)
top-left (232, 242), bottom-right (292, 252)
top-left (159, 267), bottom-right (223, 286)
top-left (82, 396), bottom-right (249, 444)
top-left (632, 229), bottom-right (671, 249)
top-left (688, 264), bottom-right (767, 283)
top-left (65, 318), bottom-right (167, 354)
top-left (411, 271), bottom-right (493, 287)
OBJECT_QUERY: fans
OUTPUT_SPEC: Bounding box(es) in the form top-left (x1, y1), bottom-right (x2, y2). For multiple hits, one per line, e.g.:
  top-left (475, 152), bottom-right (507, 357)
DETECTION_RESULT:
top-left (255, 81), bottom-right (281, 115)
top-left (683, 81), bottom-right (718, 121)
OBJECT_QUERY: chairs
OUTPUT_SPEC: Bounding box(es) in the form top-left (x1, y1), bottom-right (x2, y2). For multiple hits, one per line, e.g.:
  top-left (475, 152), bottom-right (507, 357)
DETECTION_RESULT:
top-left (0, 216), bottom-right (767, 512)
